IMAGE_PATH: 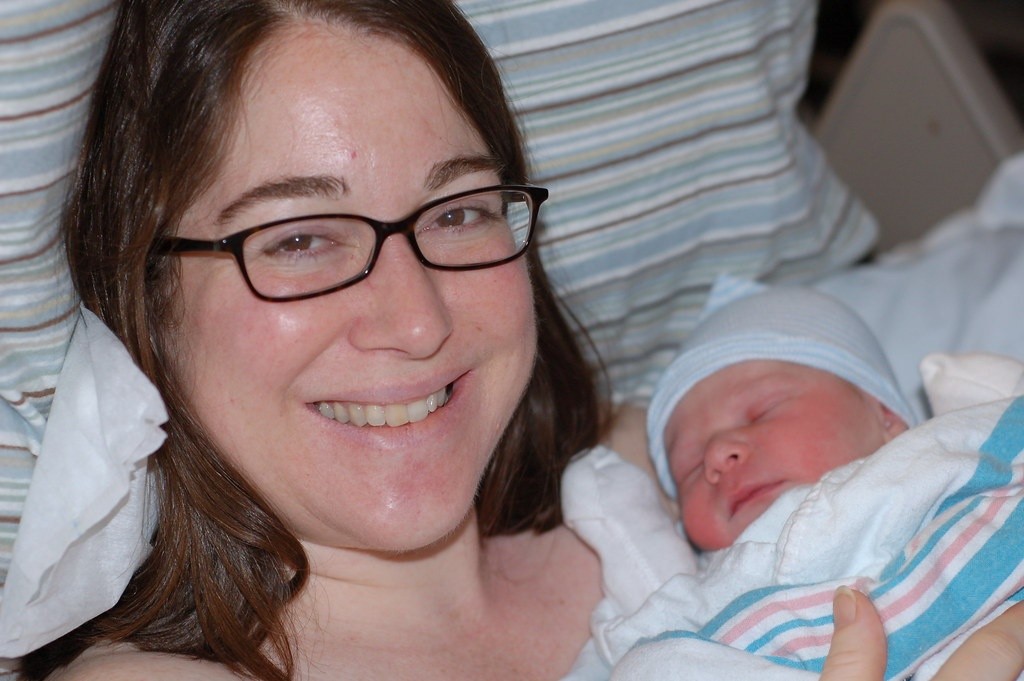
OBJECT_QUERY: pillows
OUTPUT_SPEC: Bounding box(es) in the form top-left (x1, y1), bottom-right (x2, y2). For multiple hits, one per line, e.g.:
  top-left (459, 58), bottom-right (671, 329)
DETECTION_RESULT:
top-left (0, 0), bottom-right (888, 585)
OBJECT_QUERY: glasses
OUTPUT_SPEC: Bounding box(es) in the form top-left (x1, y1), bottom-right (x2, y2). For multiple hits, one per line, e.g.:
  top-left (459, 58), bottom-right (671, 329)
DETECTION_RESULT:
top-left (148, 185), bottom-right (549, 302)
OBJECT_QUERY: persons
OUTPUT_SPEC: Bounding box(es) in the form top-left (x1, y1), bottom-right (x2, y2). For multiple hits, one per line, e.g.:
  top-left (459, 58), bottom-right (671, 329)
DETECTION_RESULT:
top-left (26, 0), bottom-right (1023, 679)
top-left (559, 280), bottom-right (1023, 681)
